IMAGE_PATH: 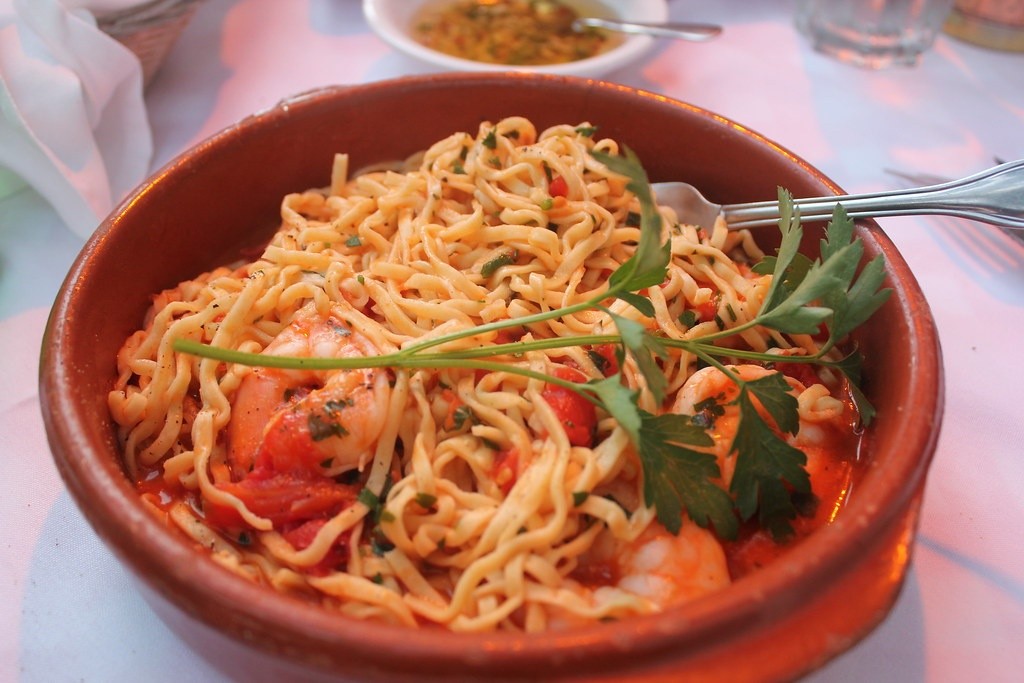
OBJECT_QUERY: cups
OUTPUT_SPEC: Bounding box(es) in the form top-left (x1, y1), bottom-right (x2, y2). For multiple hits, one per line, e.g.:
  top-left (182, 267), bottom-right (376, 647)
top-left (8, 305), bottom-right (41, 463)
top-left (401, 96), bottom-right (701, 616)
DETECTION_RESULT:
top-left (792, 0), bottom-right (953, 71)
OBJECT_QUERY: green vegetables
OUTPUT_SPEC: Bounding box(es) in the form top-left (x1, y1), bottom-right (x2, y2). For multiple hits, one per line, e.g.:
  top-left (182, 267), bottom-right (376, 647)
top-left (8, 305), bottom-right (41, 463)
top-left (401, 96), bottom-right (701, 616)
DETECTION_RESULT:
top-left (173, 148), bottom-right (889, 536)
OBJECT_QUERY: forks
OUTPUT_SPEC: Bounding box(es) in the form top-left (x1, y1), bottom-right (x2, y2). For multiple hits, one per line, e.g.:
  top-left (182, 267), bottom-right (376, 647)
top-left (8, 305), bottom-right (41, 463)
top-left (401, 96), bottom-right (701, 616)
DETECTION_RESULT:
top-left (649, 158), bottom-right (1024, 231)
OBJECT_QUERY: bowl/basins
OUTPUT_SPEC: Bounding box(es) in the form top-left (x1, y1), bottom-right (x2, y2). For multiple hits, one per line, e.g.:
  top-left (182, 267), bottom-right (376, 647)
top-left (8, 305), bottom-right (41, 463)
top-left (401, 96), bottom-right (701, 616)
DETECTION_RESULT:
top-left (0, 0), bottom-right (195, 194)
top-left (40, 71), bottom-right (944, 683)
top-left (362, 0), bottom-right (669, 79)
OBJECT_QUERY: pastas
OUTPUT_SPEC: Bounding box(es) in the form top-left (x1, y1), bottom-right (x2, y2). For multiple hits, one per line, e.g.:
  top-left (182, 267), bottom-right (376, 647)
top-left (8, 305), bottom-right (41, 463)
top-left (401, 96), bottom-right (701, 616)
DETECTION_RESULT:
top-left (107, 123), bottom-right (846, 633)
